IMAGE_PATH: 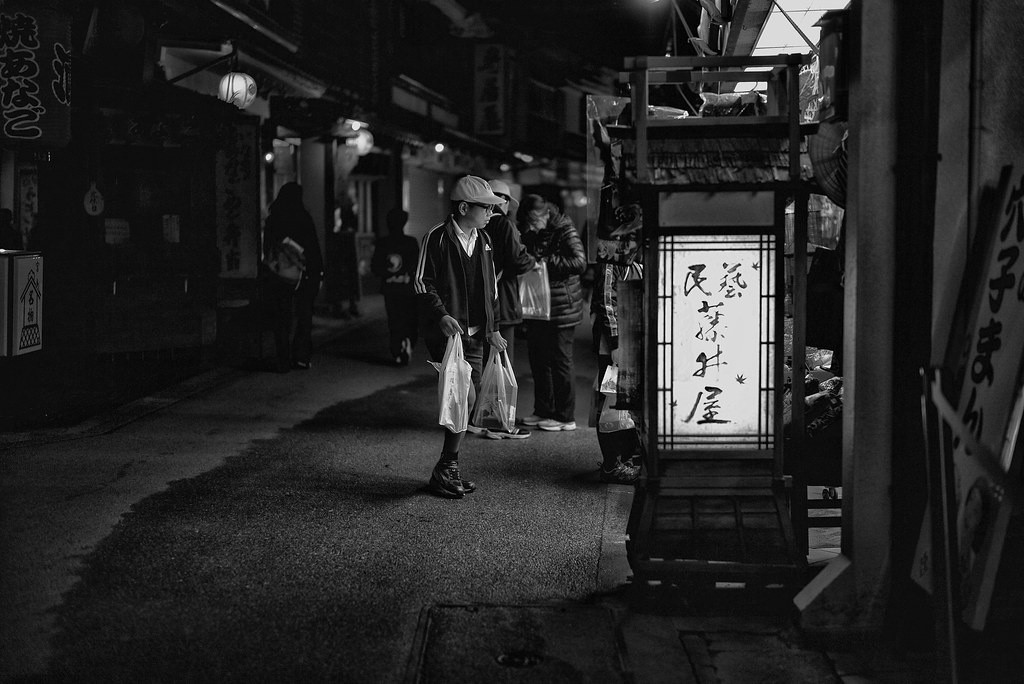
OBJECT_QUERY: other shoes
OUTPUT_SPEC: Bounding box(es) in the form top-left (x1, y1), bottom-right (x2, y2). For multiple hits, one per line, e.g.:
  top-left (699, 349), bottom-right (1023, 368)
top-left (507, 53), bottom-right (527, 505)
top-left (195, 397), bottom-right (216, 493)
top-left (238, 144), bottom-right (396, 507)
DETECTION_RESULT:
top-left (293, 358), bottom-right (312, 369)
top-left (597, 461), bottom-right (639, 483)
top-left (400, 337), bottom-right (412, 364)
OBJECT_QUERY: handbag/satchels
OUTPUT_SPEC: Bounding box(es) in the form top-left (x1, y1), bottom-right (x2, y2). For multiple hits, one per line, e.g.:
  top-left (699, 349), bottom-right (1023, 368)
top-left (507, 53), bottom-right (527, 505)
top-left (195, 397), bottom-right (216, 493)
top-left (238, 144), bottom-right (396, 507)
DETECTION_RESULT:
top-left (472, 344), bottom-right (518, 431)
top-left (427, 331), bottom-right (473, 434)
top-left (599, 363), bottom-right (618, 395)
top-left (274, 237), bottom-right (306, 290)
top-left (599, 395), bottom-right (635, 433)
top-left (517, 260), bottom-right (551, 321)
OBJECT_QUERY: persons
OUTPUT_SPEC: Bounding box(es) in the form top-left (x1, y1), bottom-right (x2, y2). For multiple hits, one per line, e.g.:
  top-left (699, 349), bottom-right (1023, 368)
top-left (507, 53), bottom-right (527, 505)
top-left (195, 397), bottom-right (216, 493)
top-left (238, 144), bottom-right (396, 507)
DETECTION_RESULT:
top-left (368, 209), bottom-right (421, 365)
top-left (412, 174), bottom-right (644, 497)
top-left (0, 208), bottom-right (23, 250)
top-left (263, 182), bottom-right (327, 374)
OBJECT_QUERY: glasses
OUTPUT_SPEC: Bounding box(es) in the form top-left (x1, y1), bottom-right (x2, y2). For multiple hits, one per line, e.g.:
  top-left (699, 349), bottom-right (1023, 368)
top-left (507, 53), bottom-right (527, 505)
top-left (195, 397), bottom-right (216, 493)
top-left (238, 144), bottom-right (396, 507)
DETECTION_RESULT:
top-left (469, 203), bottom-right (495, 213)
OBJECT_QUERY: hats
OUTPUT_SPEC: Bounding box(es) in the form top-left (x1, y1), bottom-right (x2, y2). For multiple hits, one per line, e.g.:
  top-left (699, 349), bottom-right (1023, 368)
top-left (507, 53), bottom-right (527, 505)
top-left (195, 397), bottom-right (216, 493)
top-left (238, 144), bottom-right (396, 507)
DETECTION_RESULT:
top-left (488, 180), bottom-right (519, 211)
top-left (450, 175), bottom-right (507, 204)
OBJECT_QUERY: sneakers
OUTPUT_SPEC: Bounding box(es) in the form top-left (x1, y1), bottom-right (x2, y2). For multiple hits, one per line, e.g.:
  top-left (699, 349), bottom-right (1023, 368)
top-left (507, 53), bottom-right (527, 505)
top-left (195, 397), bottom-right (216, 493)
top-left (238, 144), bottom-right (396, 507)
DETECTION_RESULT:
top-left (522, 414), bottom-right (544, 425)
top-left (485, 428), bottom-right (532, 440)
top-left (537, 418), bottom-right (577, 431)
top-left (429, 473), bottom-right (477, 493)
top-left (433, 451), bottom-right (466, 497)
top-left (467, 421), bottom-right (486, 434)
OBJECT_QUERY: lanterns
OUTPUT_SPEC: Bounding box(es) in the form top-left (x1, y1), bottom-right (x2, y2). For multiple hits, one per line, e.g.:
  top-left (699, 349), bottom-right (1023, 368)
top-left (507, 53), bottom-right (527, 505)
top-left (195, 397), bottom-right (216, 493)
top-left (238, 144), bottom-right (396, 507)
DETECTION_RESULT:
top-left (219, 70), bottom-right (257, 109)
top-left (346, 130), bottom-right (374, 155)
top-left (0, 0), bottom-right (73, 162)
